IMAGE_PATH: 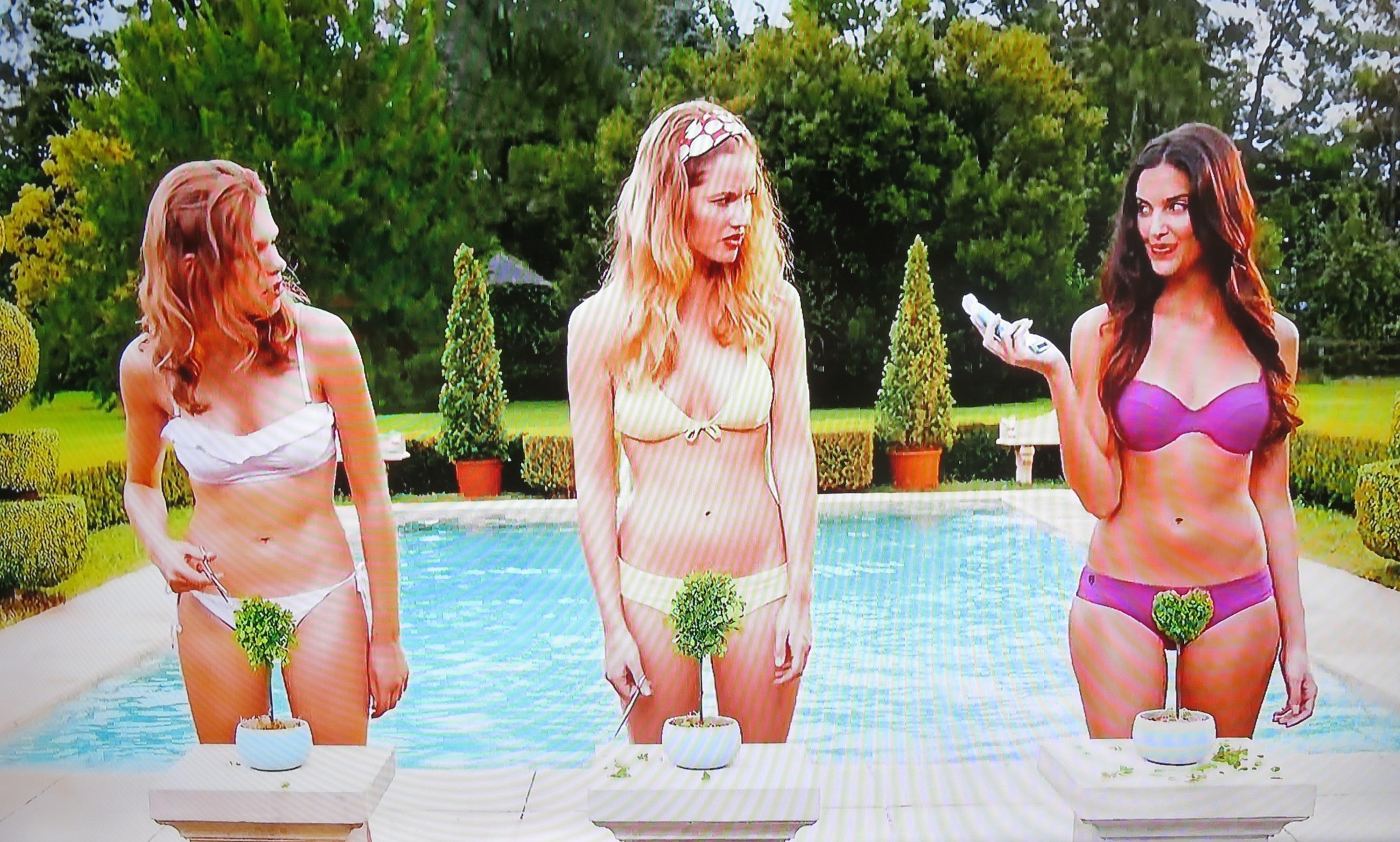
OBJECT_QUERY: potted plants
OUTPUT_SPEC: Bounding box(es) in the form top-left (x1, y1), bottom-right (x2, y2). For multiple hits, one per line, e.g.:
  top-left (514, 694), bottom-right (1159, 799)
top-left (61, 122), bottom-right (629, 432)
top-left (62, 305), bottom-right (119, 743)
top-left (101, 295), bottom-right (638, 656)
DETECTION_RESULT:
top-left (437, 244), bottom-right (510, 498)
top-left (661, 569), bottom-right (746, 770)
top-left (873, 234), bottom-right (955, 489)
top-left (1132, 588), bottom-right (1219, 765)
top-left (232, 596), bottom-right (314, 772)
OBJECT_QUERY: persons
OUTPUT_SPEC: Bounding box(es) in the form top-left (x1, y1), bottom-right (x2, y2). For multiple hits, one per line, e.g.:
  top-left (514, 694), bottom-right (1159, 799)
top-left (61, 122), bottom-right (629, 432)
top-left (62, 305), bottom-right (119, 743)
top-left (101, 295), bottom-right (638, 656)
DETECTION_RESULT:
top-left (567, 100), bottom-right (819, 745)
top-left (981, 123), bottom-right (1318, 745)
top-left (118, 160), bottom-right (410, 744)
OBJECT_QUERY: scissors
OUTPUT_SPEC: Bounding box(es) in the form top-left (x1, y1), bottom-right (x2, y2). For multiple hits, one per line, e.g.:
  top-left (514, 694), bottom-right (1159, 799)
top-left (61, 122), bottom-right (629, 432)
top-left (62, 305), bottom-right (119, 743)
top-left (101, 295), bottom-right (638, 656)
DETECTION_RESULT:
top-left (614, 675), bottom-right (646, 737)
top-left (197, 546), bottom-right (228, 603)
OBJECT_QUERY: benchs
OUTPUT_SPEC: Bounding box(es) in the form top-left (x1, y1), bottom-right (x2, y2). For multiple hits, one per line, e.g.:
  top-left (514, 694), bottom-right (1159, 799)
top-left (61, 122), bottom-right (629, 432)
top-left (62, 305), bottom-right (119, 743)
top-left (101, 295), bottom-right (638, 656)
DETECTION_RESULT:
top-left (996, 408), bottom-right (1062, 483)
